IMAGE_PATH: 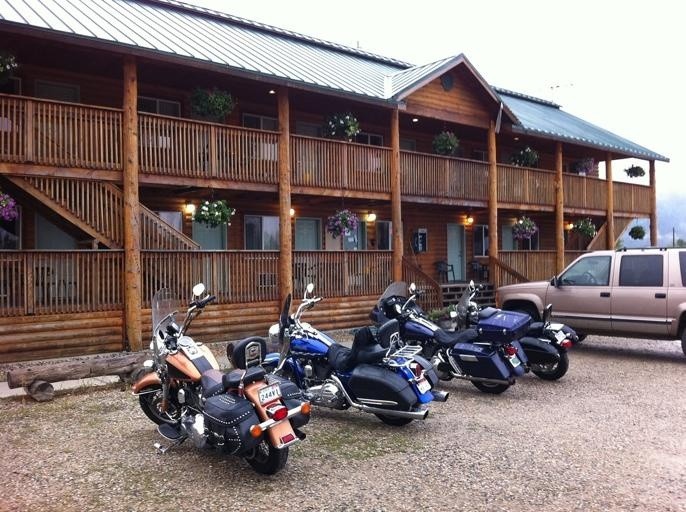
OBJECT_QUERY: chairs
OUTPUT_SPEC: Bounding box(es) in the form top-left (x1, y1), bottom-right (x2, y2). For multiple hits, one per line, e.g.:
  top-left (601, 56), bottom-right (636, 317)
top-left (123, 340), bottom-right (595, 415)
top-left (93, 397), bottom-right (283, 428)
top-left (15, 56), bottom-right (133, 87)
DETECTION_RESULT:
top-left (434, 261), bottom-right (456, 283)
top-left (468, 261), bottom-right (488, 280)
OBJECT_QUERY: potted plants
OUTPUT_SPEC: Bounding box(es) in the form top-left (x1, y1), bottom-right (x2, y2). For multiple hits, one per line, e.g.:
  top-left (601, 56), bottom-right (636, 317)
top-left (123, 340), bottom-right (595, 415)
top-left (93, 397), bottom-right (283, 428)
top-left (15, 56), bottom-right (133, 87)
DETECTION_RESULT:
top-left (428, 306), bottom-right (458, 328)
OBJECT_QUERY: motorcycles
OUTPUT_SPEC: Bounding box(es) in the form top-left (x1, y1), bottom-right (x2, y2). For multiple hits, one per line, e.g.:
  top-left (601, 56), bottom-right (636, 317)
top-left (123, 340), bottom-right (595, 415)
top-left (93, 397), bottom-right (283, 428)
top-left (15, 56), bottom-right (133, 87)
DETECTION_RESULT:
top-left (361, 274), bottom-right (534, 396)
top-left (434, 282), bottom-right (580, 383)
top-left (258, 279), bottom-right (450, 428)
top-left (128, 283), bottom-right (313, 475)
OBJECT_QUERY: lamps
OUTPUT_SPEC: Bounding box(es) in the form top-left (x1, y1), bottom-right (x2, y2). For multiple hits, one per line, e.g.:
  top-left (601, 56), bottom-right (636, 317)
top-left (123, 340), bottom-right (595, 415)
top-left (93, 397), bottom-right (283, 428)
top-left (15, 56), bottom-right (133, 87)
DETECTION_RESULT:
top-left (366, 210), bottom-right (377, 223)
top-left (184, 199), bottom-right (195, 219)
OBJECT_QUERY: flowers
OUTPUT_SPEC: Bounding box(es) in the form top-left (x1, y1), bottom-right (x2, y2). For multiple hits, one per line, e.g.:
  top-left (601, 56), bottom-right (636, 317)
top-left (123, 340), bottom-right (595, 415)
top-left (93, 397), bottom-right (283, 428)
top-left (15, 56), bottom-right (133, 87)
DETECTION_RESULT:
top-left (512, 217), bottom-right (598, 240)
top-left (0, 54), bottom-right (17, 74)
top-left (0, 191), bottom-right (18, 222)
top-left (196, 199), bottom-right (236, 227)
top-left (326, 210), bottom-right (359, 239)
top-left (431, 132), bottom-right (460, 155)
top-left (326, 112), bottom-right (361, 139)
top-left (506, 146), bottom-right (595, 178)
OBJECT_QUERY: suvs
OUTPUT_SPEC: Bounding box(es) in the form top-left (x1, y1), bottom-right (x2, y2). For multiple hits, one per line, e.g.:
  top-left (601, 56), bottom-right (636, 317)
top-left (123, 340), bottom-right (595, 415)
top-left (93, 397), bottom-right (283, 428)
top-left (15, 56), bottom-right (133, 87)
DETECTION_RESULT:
top-left (494, 247), bottom-right (686, 356)
